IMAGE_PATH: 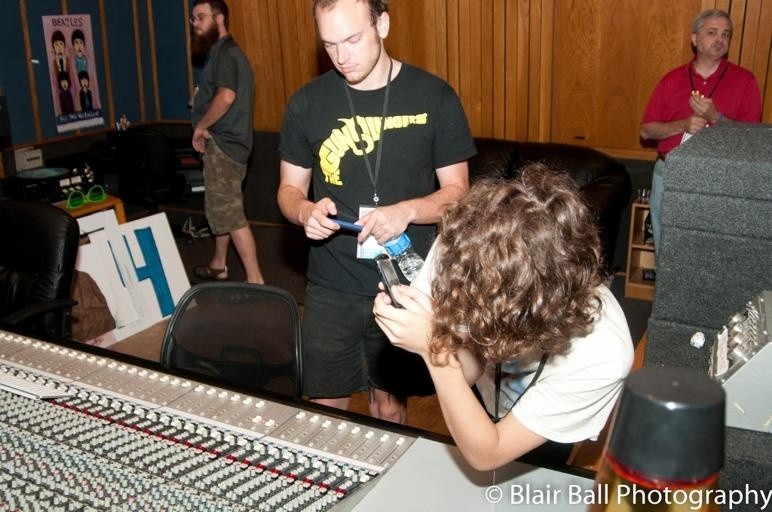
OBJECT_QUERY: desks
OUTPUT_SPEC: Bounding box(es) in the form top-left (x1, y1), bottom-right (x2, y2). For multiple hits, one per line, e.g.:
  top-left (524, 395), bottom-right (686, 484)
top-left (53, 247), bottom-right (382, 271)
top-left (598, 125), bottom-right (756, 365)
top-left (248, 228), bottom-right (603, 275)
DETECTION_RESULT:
top-left (52, 192), bottom-right (127, 228)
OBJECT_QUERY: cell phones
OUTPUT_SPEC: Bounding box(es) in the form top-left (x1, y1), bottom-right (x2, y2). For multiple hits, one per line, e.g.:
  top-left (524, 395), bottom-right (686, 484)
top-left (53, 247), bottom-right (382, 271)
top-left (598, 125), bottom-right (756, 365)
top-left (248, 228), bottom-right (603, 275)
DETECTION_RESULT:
top-left (329, 218), bottom-right (363, 233)
top-left (374, 253), bottom-right (404, 309)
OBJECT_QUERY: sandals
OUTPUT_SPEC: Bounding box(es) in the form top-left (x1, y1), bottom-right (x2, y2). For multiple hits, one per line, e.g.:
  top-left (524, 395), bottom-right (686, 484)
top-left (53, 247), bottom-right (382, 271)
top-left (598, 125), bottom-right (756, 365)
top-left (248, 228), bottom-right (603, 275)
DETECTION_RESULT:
top-left (193, 264), bottom-right (229, 281)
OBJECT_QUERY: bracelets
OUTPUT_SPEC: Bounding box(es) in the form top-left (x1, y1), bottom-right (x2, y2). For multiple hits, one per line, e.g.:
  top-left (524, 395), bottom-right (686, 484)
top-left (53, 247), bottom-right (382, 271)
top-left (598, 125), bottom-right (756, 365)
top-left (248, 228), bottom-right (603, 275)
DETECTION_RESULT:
top-left (717, 113), bottom-right (727, 121)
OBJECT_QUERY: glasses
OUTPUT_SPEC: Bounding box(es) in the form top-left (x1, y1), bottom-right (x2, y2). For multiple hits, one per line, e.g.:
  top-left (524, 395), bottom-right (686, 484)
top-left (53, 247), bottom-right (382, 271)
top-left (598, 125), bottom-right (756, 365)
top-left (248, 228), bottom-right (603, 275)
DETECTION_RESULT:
top-left (189, 14), bottom-right (216, 24)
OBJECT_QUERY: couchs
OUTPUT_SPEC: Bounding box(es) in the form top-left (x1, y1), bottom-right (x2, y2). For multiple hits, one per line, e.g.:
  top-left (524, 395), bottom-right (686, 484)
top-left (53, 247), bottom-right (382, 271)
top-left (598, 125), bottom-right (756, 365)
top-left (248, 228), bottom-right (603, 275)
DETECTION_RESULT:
top-left (87, 118), bottom-right (301, 233)
top-left (466, 131), bottom-right (625, 276)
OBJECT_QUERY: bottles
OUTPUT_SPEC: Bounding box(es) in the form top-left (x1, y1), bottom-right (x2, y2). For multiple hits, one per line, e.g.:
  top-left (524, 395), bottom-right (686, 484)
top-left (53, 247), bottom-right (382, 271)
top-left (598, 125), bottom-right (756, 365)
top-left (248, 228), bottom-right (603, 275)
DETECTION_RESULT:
top-left (382, 233), bottom-right (424, 284)
top-left (586, 366), bottom-right (727, 510)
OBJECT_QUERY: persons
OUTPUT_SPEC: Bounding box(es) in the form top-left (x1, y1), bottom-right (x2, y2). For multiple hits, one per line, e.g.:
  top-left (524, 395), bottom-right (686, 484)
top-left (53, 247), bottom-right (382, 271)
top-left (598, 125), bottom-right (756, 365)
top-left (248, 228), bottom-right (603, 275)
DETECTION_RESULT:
top-left (57, 70), bottom-right (75, 116)
top-left (371, 162), bottom-right (635, 472)
top-left (277, 0), bottom-right (480, 426)
top-left (51, 30), bottom-right (71, 75)
top-left (189, 1), bottom-right (268, 284)
top-left (71, 29), bottom-right (88, 77)
top-left (79, 70), bottom-right (94, 112)
top-left (637, 8), bottom-right (762, 273)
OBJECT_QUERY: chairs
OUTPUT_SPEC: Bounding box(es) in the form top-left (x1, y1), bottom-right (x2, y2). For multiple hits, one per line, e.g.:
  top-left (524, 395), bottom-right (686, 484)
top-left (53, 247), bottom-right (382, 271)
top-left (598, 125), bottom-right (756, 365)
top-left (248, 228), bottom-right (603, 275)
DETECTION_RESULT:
top-left (0, 190), bottom-right (80, 344)
top-left (157, 279), bottom-right (307, 403)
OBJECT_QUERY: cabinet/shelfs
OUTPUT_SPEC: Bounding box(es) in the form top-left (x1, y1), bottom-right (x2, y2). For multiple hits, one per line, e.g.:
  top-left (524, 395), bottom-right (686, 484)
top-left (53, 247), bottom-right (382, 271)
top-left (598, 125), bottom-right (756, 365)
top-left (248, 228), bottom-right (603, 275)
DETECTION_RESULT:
top-left (622, 193), bottom-right (661, 303)
